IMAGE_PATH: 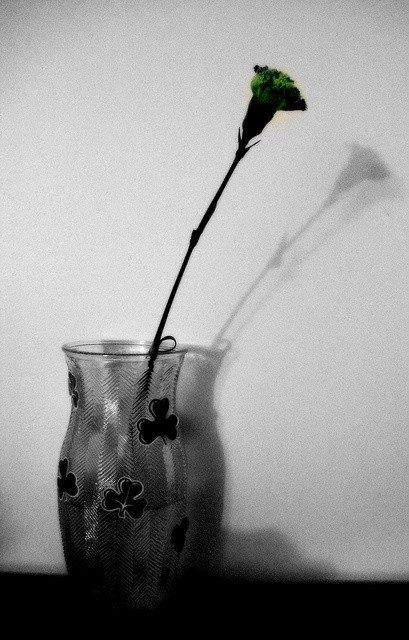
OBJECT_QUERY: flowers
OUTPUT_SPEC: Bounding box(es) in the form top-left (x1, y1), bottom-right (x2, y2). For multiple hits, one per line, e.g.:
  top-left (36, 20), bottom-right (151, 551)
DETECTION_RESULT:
top-left (122, 61), bottom-right (307, 447)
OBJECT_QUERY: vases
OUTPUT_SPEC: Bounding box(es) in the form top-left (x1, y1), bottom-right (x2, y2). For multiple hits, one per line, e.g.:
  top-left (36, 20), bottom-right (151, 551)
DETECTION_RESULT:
top-left (59, 341), bottom-right (189, 606)
